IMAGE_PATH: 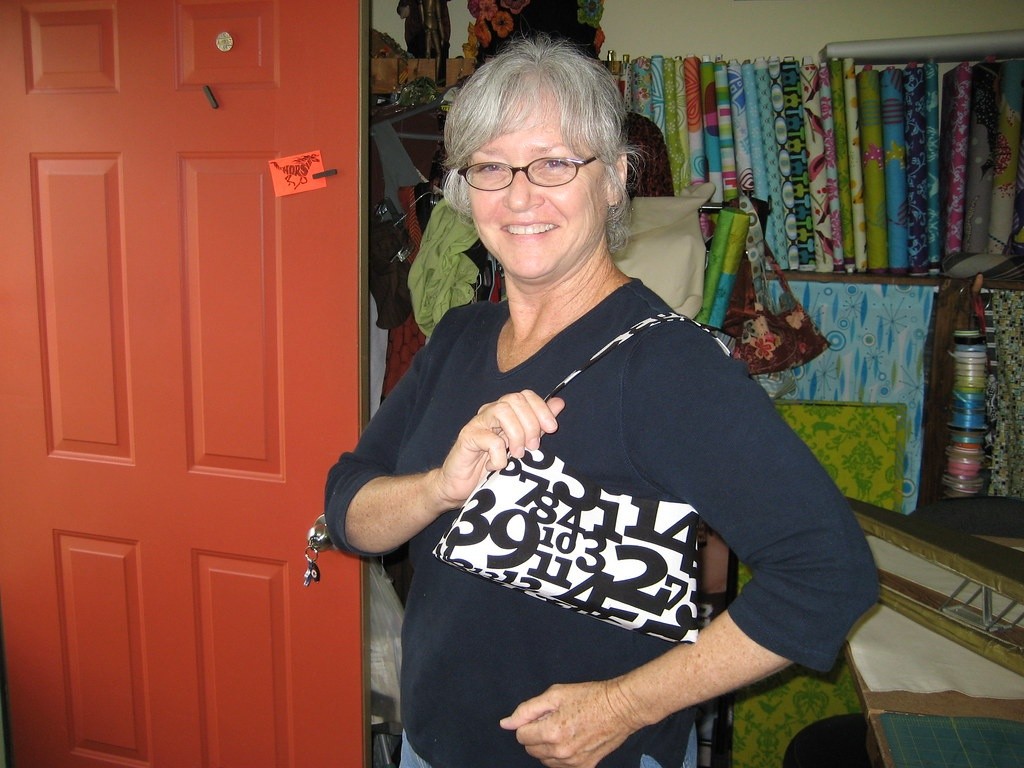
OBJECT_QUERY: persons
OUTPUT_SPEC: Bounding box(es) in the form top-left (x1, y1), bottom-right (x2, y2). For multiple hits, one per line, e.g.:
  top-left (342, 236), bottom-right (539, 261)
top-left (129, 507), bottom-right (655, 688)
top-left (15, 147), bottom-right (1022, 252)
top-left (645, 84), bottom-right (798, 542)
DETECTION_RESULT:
top-left (325, 44), bottom-right (879, 768)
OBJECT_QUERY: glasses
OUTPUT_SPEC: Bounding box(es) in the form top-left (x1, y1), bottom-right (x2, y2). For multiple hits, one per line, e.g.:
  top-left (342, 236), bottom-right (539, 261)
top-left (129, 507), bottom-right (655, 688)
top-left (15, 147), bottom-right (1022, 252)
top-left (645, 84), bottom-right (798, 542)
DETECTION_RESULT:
top-left (458, 156), bottom-right (603, 192)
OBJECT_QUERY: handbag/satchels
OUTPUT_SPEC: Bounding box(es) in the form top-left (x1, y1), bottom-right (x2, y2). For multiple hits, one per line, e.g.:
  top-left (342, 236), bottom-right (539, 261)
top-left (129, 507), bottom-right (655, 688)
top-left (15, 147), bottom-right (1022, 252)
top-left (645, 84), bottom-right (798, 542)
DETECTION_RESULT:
top-left (432, 312), bottom-right (734, 644)
top-left (732, 240), bottom-right (831, 376)
